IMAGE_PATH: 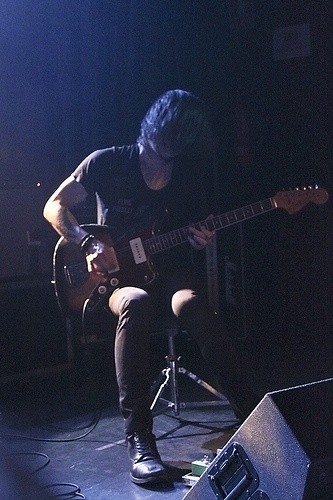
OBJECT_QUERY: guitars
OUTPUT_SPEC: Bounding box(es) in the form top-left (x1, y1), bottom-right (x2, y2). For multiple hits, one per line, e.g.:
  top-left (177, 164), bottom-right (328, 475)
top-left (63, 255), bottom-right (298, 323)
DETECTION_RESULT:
top-left (51, 185), bottom-right (329, 324)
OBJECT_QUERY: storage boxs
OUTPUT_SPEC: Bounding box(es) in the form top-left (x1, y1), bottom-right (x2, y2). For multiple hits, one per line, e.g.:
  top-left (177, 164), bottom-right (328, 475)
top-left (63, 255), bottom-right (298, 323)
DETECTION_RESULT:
top-left (0, 272), bottom-right (68, 401)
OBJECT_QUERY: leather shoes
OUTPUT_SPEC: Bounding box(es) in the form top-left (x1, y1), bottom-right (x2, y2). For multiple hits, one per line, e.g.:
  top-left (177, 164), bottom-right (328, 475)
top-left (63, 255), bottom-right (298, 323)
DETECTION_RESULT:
top-left (125, 433), bottom-right (167, 485)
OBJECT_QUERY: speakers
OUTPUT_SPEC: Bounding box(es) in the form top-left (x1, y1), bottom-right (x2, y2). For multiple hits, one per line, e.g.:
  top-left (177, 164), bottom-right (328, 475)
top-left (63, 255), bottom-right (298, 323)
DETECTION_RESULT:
top-left (183, 377), bottom-right (332, 500)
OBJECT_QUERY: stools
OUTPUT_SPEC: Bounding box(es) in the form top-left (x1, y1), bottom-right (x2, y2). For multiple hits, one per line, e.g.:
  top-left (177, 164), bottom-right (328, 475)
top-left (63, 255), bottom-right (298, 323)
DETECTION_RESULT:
top-left (148, 313), bottom-right (234, 413)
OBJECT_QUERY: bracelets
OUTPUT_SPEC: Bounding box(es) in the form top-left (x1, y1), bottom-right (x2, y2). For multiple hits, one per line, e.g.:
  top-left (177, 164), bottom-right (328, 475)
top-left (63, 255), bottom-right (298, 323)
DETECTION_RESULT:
top-left (79, 233), bottom-right (95, 249)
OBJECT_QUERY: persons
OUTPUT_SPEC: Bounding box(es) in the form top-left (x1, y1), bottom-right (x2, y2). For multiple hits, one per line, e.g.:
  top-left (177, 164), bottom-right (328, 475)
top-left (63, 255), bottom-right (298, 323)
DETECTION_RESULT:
top-left (42, 89), bottom-right (263, 485)
